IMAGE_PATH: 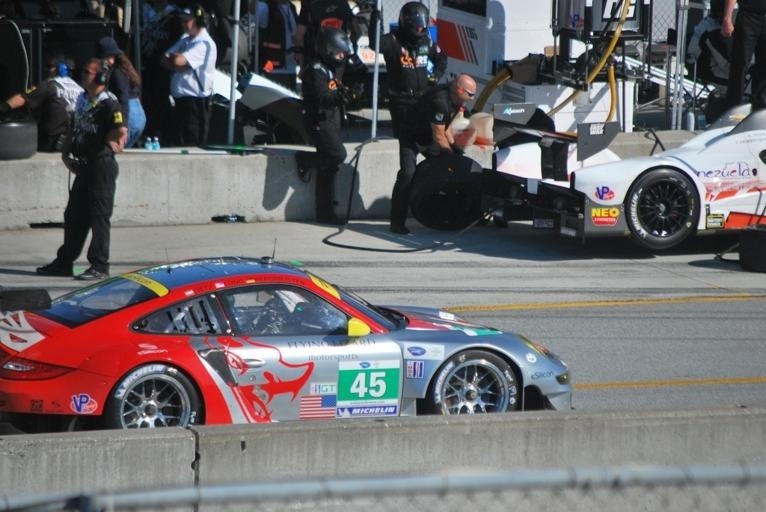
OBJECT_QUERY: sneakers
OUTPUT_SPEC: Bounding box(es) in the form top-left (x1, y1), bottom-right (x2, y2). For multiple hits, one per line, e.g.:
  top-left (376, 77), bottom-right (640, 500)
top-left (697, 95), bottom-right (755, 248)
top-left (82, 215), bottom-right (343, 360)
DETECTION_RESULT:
top-left (294, 149), bottom-right (312, 185)
top-left (315, 211), bottom-right (348, 226)
top-left (74, 265), bottom-right (110, 281)
top-left (390, 223), bottom-right (410, 235)
top-left (35, 260), bottom-right (74, 277)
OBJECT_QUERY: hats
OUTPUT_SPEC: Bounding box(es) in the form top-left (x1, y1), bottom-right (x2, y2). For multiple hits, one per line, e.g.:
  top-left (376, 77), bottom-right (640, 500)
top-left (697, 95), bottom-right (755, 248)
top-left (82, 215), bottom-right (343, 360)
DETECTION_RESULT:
top-left (94, 36), bottom-right (123, 60)
top-left (176, 3), bottom-right (207, 23)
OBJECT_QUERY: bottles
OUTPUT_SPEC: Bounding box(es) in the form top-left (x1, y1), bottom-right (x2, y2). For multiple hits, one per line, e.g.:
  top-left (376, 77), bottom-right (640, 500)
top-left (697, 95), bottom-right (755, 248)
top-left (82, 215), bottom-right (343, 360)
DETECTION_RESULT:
top-left (144, 136), bottom-right (161, 151)
top-left (211, 214), bottom-right (245, 222)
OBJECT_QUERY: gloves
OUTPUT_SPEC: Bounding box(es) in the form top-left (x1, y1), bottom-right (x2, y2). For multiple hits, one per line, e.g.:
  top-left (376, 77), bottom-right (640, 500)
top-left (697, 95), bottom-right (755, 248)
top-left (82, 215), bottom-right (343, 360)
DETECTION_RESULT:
top-left (370, 3), bottom-right (384, 33)
top-left (60, 140), bottom-right (115, 170)
top-left (449, 143), bottom-right (465, 158)
top-left (0, 101), bottom-right (12, 120)
top-left (427, 72), bottom-right (441, 86)
top-left (339, 80), bottom-right (365, 104)
top-left (440, 147), bottom-right (458, 161)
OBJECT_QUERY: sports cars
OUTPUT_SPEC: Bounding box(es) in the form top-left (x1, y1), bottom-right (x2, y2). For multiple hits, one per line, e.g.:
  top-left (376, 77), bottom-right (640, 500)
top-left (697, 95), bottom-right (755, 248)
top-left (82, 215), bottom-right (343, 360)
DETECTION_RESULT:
top-left (409, 98), bottom-right (766, 257)
top-left (0, 252), bottom-right (576, 435)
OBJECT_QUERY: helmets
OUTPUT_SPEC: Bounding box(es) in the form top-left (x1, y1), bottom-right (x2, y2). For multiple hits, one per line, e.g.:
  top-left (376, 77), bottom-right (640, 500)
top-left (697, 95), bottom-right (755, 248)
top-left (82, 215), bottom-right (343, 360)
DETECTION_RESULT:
top-left (313, 25), bottom-right (351, 69)
top-left (397, 2), bottom-right (430, 48)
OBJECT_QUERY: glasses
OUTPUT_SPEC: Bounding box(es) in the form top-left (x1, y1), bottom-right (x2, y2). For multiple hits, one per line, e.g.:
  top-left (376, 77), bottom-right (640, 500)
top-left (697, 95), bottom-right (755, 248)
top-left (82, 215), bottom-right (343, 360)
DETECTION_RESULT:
top-left (461, 88), bottom-right (477, 99)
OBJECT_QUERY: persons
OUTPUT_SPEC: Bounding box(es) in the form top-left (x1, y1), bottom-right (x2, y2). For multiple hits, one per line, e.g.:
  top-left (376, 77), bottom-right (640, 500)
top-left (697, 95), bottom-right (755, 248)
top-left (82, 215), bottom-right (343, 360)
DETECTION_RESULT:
top-left (0, 1), bottom-right (386, 153)
top-left (390, 75), bottom-right (476, 235)
top-left (685, 0), bottom-right (754, 103)
top-left (37, 59), bottom-right (128, 281)
top-left (299, 28), bottom-right (365, 224)
top-left (719, 0), bottom-right (766, 113)
top-left (368, 2), bottom-right (448, 138)
